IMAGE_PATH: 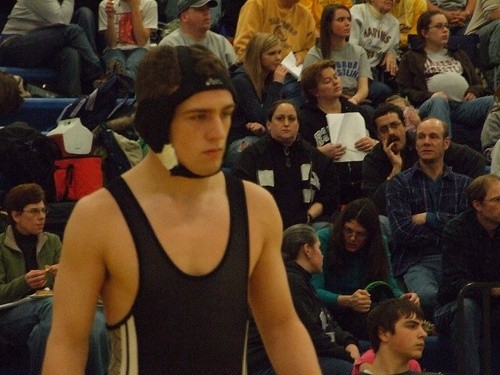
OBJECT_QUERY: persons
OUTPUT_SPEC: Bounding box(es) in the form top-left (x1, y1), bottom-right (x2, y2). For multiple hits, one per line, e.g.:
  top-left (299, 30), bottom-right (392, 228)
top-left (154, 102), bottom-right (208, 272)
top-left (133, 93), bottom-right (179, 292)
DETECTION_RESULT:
top-left (0, 0), bottom-right (500, 375)
top-left (41, 45), bottom-right (323, 375)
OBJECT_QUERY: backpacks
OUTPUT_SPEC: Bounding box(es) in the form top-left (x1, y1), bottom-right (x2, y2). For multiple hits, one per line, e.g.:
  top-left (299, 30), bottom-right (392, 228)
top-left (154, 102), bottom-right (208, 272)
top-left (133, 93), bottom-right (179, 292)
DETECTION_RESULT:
top-left (58, 74), bottom-right (137, 129)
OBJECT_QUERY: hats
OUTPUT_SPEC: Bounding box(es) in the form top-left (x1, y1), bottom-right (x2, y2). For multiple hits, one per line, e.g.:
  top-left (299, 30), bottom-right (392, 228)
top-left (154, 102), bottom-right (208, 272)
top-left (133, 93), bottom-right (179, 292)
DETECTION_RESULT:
top-left (177, 0), bottom-right (218, 18)
top-left (132, 44), bottom-right (236, 179)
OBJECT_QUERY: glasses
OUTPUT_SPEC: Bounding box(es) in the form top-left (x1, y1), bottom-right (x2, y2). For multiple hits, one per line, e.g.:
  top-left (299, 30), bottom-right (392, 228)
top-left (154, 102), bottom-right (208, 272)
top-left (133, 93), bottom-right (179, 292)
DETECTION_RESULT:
top-left (478, 196), bottom-right (500, 202)
top-left (377, 121), bottom-right (403, 134)
top-left (427, 22), bottom-right (450, 30)
top-left (23, 208), bottom-right (48, 216)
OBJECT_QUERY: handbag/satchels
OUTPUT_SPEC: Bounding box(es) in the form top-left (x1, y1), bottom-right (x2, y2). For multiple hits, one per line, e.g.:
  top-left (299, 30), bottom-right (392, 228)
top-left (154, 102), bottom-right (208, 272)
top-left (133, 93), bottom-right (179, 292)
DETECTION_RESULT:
top-left (48, 155), bottom-right (105, 200)
